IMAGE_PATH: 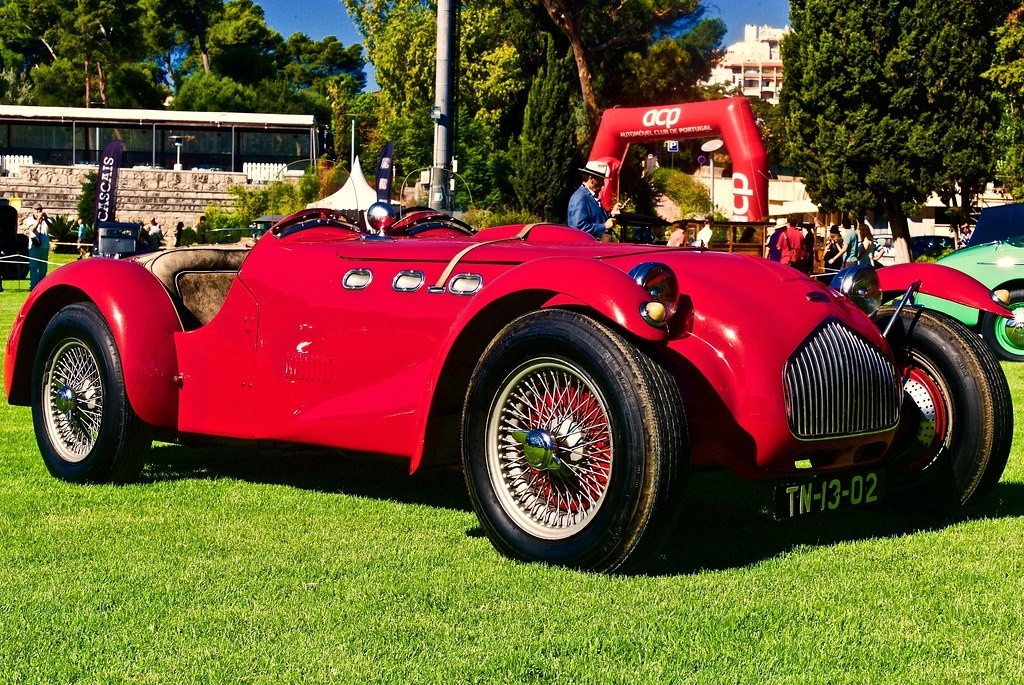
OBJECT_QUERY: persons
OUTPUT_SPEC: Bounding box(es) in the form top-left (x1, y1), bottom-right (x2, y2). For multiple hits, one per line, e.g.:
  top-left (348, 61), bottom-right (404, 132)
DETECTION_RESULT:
top-left (28, 203), bottom-right (56, 291)
top-left (144, 215), bottom-right (208, 257)
top-left (566, 159), bottom-right (625, 242)
top-left (77, 218), bottom-right (88, 261)
top-left (957, 223), bottom-right (973, 248)
top-left (631, 214), bottom-right (911, 287)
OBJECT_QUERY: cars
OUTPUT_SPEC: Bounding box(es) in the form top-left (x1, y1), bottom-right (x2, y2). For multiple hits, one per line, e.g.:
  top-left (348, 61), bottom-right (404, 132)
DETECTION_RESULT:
top-left (906, 239), bottom-right (1024, 362)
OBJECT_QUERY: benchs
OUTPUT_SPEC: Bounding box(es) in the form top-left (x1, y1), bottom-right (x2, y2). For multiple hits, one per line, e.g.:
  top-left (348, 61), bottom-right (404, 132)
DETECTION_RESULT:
top-left (130, 246), bottom-right (253, 332)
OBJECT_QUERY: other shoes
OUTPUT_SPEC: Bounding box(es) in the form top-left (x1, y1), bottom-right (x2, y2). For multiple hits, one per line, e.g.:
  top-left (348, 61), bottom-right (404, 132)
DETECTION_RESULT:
top-left (86, 252), bottom-right (90, 259)
top-left (77, 257), bottom-right (83, 260)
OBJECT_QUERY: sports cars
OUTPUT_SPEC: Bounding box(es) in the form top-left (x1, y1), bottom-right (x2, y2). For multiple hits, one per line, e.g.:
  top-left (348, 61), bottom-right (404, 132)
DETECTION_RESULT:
top-left (4, 159), bottom-right (1012, 576)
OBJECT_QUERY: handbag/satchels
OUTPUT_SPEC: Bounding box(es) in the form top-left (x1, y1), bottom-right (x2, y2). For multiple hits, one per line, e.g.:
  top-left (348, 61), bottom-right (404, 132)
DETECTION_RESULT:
top-left (788, 249), bottom-right (809, 268)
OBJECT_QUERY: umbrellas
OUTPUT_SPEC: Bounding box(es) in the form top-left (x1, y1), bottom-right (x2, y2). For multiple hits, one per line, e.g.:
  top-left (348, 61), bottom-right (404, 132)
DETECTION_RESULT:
top-left (303, 154), bottom-right (402, 227)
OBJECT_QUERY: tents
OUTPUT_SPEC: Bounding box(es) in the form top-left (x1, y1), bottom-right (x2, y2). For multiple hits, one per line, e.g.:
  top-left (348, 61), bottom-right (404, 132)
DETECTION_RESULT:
top-left (962, 201), bottom-right (1024, 247)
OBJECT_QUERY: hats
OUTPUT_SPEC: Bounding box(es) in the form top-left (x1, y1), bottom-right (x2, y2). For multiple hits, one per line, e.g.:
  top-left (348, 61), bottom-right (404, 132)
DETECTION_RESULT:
top-left (579, 161), bottom-right (608, 178)
top-left (33, 203), bottom-right (43, 209)
top-left (830, 225), bottom-right (841, 233)
